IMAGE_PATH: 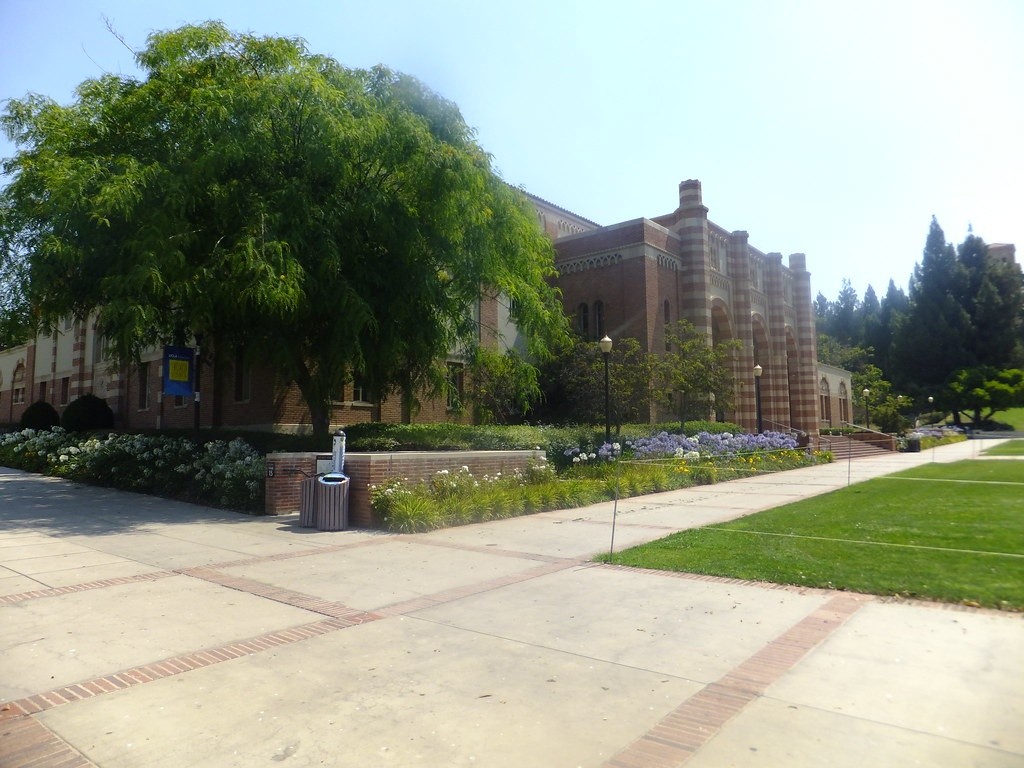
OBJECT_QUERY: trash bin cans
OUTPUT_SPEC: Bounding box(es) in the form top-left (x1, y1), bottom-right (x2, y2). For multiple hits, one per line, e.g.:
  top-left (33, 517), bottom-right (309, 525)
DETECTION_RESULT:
top-left (907, 439), bottom-right (920, 452)
top-left (316, 472), bottom-right (350, 532)
top-left (298, 477), bottom-right (316, 528)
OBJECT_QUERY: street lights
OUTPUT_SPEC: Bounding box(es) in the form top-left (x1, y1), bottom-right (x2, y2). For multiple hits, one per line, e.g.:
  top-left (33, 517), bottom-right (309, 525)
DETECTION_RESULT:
top-left (753, 364), bottom-right (763, 433)
top-left (862, 388), bottom-right (870, 429)
top-left (599, 334), bottom-right (613, 445)
top-left (928, 397), bottom-right (934, 428)
